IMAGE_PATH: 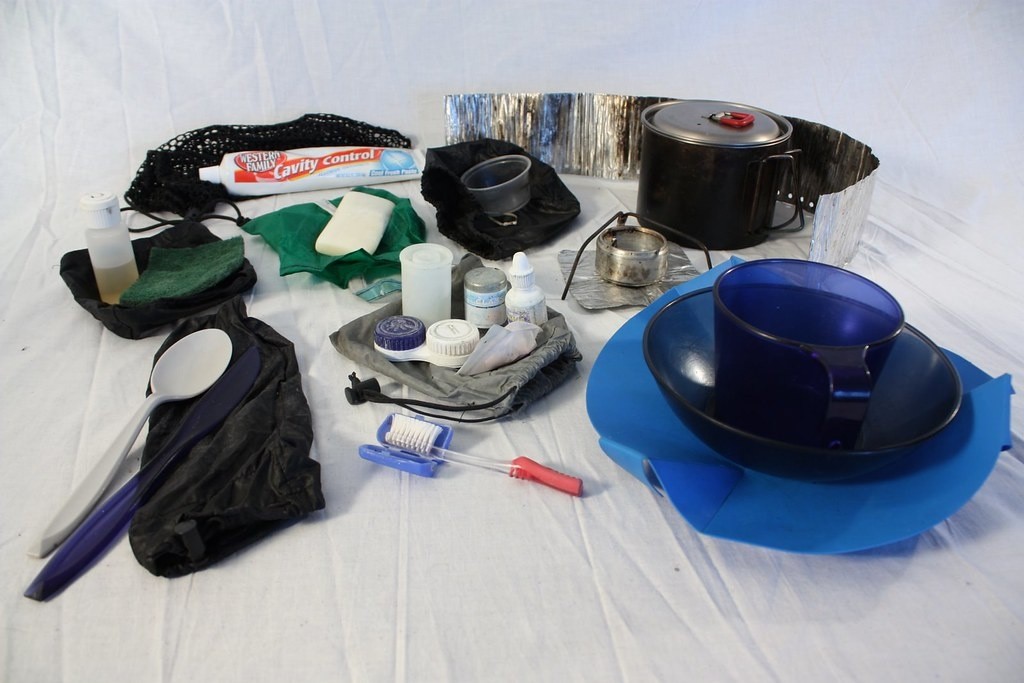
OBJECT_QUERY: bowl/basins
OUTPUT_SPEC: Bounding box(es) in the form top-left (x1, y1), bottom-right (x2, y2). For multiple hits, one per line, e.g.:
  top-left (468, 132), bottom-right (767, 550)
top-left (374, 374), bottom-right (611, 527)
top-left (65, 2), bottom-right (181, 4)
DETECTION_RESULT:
top-left (461, 155), bottom-right (532, 217)
top-left (595, 225), bottom-right (668, 287)
top-left (643, 286), bottom-right (963, 482)
top-left (711, 258), bottom-right (905, 444)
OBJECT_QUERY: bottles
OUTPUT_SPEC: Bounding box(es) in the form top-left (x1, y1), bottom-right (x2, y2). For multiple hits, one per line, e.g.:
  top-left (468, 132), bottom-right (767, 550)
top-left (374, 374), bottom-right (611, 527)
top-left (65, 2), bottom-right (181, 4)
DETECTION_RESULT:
top-left (505, 252), bottom-right (547, 326)
top-left (81, 190), bottom-right (139, 304)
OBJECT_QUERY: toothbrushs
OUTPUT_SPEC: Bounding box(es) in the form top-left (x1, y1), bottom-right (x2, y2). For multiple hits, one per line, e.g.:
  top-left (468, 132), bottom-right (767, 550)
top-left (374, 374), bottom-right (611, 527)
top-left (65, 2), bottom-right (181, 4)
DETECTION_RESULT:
top-left (382, 412), bottom-right (585, 497)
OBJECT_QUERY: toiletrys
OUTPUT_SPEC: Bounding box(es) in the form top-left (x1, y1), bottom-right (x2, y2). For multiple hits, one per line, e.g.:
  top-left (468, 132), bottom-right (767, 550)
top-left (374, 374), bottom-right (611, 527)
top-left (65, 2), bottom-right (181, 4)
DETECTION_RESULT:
top-left (198, 146), bottom-right (426, 197)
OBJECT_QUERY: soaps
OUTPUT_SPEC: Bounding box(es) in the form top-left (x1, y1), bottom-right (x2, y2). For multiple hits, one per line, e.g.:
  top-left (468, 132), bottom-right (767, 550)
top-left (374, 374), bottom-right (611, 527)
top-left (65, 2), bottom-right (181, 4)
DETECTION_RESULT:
top-left (314, 191), bottom-right (395, 257)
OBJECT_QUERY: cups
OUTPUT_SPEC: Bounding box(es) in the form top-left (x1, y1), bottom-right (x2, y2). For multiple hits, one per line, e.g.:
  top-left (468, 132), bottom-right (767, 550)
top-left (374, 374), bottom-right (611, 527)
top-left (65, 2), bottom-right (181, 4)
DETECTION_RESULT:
top-left (399, 243), bottom-right (453, 330)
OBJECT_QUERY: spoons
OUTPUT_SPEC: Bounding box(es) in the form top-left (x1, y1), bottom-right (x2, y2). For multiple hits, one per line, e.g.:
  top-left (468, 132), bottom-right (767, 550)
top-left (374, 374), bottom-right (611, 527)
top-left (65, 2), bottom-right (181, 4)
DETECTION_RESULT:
top-left (28, 328), bottom-right (232, 560)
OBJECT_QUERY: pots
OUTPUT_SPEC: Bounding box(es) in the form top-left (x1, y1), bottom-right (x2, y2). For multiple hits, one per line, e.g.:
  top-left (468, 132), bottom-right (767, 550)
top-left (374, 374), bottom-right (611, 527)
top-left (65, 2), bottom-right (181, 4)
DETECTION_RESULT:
top-left (636, 100), bottom-right (805, 251)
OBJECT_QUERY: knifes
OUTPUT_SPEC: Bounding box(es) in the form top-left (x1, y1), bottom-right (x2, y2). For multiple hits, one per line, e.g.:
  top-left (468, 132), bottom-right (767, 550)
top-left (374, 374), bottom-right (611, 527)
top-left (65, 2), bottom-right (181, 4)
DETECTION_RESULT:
top-left (24, 346), bottom-right (260, 602)
